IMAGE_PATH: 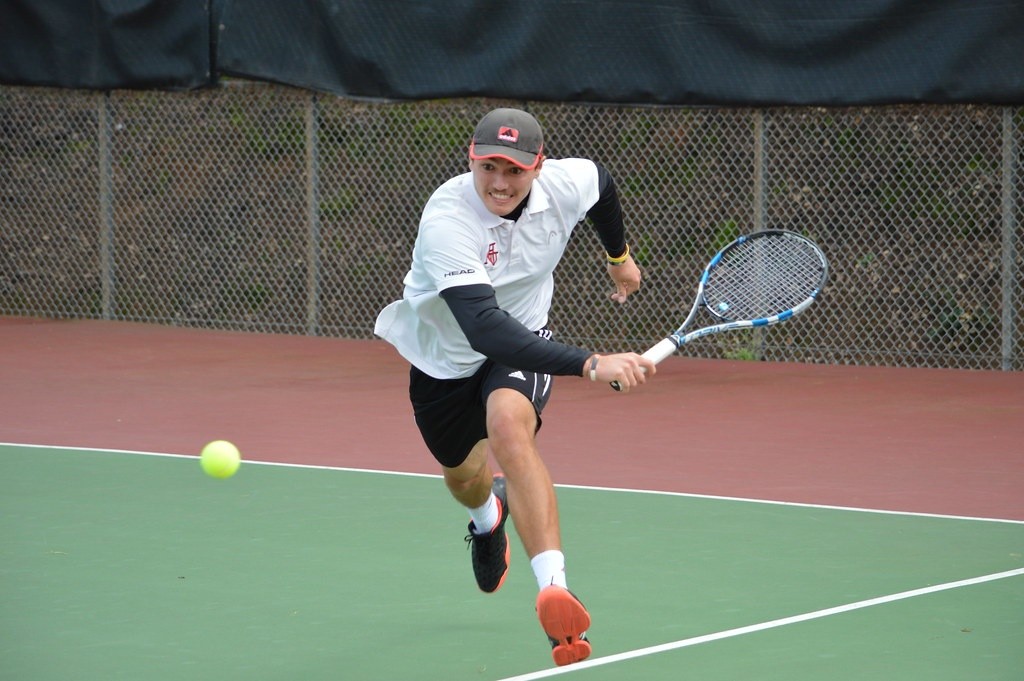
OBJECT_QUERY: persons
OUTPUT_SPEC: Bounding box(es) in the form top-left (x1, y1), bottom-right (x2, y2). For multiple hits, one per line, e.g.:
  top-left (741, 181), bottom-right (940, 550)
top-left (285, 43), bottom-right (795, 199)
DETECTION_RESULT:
top-left (374, 108), bottom-right (656, 667)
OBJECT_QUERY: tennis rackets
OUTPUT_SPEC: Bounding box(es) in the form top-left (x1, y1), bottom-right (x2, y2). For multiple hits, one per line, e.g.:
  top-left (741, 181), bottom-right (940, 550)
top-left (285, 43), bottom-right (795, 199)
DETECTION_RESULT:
top-left (609, 226), bottom-right (830, 393)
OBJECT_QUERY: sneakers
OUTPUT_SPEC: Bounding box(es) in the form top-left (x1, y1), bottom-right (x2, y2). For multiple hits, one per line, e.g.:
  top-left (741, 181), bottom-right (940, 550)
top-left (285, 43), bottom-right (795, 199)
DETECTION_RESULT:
top-left (464, 473), bottom-right (511, 594)
top-left (537, 585), bottom-right (591, 666)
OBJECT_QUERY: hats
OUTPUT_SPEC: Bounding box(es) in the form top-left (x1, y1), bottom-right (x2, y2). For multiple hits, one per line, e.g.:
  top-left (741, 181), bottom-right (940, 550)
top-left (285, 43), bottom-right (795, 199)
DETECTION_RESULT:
top-left (470, 107), bottom-right (544, 170)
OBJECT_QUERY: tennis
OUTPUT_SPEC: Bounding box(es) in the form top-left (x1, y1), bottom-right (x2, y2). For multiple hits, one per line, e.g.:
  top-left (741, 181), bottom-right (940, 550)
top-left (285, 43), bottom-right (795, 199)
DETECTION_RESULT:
top-left (201, 440), bottom-right (240, 478)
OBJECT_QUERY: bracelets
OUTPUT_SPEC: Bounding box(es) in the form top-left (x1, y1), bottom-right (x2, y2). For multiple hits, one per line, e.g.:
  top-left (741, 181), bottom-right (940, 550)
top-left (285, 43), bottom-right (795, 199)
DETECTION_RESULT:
top-left (586, 359), bottom-right (592, 377)
top-left (608, 261), bottom-right (627, 265)
top-left (606, 244), bottom-right (629, 262)
top-left (591, 355), bottom-right (601, 382)
